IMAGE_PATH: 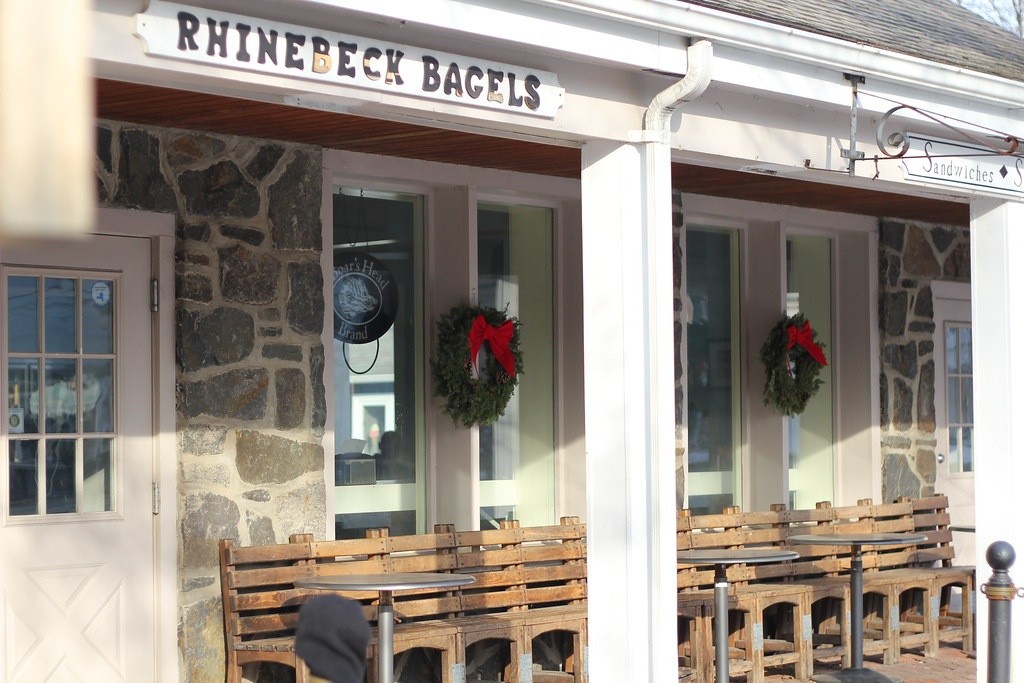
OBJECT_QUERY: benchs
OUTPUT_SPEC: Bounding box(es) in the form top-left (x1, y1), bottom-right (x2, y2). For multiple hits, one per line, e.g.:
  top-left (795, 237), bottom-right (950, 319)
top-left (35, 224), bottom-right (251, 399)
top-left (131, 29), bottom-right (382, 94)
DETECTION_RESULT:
top-left (219, 495), bottom-right (977, 683)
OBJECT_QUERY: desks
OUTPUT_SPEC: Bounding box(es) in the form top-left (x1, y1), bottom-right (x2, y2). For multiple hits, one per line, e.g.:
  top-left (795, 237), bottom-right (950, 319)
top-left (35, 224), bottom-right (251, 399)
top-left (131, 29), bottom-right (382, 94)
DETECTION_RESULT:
top-left (784, 532), bottom-right (929, 683)
top-left (675, 546), bottom-right (800, 683)
top-left (293, 574), bottom-right (475, 683)
